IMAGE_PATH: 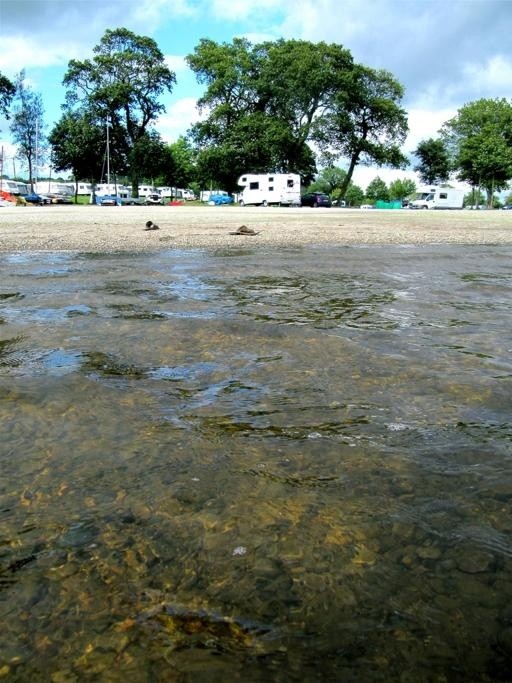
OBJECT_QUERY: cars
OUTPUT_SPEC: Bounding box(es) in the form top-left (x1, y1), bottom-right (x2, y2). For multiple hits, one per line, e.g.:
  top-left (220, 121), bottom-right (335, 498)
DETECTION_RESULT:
top-left (503, 205), bottom-right (511, 209)
top-left (37, 194), bottom-right (64, 204)
top-left (145, 194), bottom-right (165, 205)
top-left (140, 186), bottom-right (196, 200)
top-left (0, 179), bottom-right (130, 196)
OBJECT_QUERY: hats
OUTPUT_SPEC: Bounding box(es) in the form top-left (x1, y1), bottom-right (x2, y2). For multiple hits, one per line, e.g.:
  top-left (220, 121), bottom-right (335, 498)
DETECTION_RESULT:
top-left (229, 225), bottom-right (258, 235)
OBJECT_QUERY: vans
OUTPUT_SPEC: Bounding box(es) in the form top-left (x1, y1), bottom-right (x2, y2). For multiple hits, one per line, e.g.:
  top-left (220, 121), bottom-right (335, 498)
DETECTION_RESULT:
top-left (332, 200), bottom-right (346, 207)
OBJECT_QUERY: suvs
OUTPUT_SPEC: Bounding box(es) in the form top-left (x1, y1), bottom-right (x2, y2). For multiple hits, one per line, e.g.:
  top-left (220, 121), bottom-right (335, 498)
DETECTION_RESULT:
top-left (298, 191), bottom-right (332, 208)
